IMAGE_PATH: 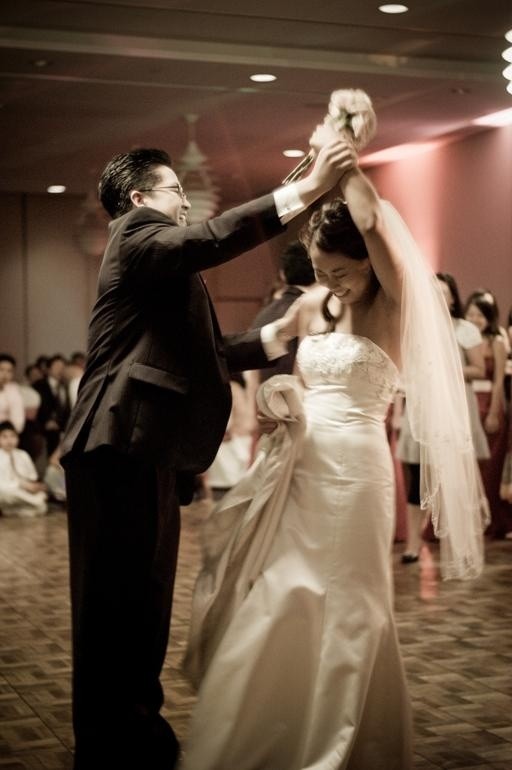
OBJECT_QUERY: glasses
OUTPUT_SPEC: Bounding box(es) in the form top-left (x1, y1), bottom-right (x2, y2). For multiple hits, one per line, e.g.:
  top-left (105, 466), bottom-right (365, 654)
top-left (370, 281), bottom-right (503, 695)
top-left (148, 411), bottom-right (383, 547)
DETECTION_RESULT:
top-left (138, 184), bottom-right (184, 199)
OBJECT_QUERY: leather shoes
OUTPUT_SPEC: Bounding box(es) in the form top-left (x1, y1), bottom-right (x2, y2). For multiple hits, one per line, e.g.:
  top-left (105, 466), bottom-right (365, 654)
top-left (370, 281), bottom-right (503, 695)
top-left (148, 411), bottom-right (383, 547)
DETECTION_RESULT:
top-left (401, 554), bottom-right (418, 563)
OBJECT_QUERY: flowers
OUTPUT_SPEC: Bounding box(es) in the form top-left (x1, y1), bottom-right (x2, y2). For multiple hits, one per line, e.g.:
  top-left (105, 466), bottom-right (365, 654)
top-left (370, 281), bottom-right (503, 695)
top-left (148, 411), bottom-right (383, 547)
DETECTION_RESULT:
top-left (280, 86), bottom-right (377, 187)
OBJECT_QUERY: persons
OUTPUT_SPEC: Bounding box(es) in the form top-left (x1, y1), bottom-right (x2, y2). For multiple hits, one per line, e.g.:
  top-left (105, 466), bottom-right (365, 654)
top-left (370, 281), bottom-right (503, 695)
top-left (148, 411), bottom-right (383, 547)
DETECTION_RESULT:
top-left (193, 241), bottom-right (316, 505)
top-left (385, 273), bottom-right (511, 566)
top-left (179, 123), bottom-right (418, 769)
top-left (57, 138), bottom-right (360, 770)
top-left (1, 352), bottom-right (90, 516)
